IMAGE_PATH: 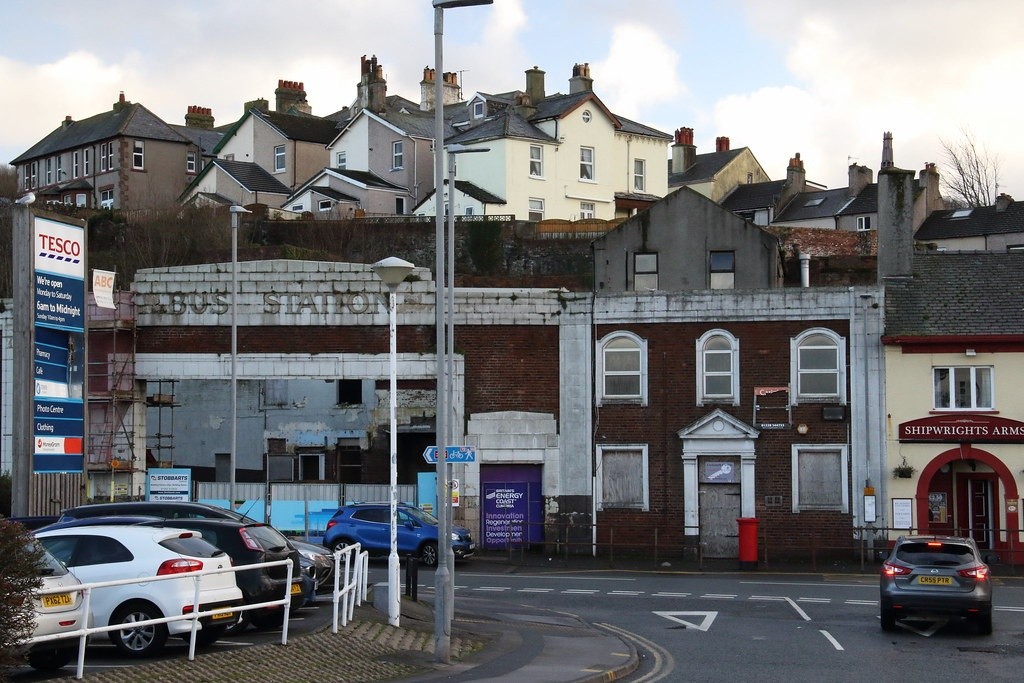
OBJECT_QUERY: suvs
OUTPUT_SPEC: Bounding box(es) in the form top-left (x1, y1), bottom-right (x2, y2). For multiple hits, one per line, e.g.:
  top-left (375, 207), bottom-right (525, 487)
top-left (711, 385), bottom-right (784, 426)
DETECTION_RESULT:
top-left (322, 500), bottom-right (475, 567)
top-left (880, 534), bottom-right (993, 634)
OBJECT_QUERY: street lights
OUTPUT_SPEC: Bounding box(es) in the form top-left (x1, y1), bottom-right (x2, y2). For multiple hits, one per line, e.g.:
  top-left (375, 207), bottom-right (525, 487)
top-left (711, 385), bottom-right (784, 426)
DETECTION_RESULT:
top-left (229, 203), bottom-right (254, 514)
top-left (445, 141), bottom-right (490, 616)
top-left (430, 0), bottom-right (490, 665)
top-left (373, 257), bottom-right (416, 629)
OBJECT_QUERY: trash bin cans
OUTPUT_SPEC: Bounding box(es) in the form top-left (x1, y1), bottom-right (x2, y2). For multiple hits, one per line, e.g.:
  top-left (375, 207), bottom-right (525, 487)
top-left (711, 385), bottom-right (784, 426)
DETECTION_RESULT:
top-left (736, 517), bottom-right (761, 562)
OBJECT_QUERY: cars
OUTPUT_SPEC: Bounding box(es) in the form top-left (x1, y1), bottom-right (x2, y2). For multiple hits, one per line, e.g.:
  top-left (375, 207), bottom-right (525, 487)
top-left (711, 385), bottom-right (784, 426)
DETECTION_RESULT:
top-left (289, 540), bottom-right (335, 594)
top-left (35, 525), bottom-right (244, 660)
top-left (133, 518), bottom-right (306, 637)
top-left (0, 536), bottom-right (94, 671)
top-left (28, 515), bottom-right (317, 615)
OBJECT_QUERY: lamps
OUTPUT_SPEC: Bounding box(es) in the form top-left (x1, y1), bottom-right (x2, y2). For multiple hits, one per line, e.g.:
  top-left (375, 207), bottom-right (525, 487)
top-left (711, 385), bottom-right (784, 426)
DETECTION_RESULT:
top-left (966, 349), bottom-right (976, 356)
